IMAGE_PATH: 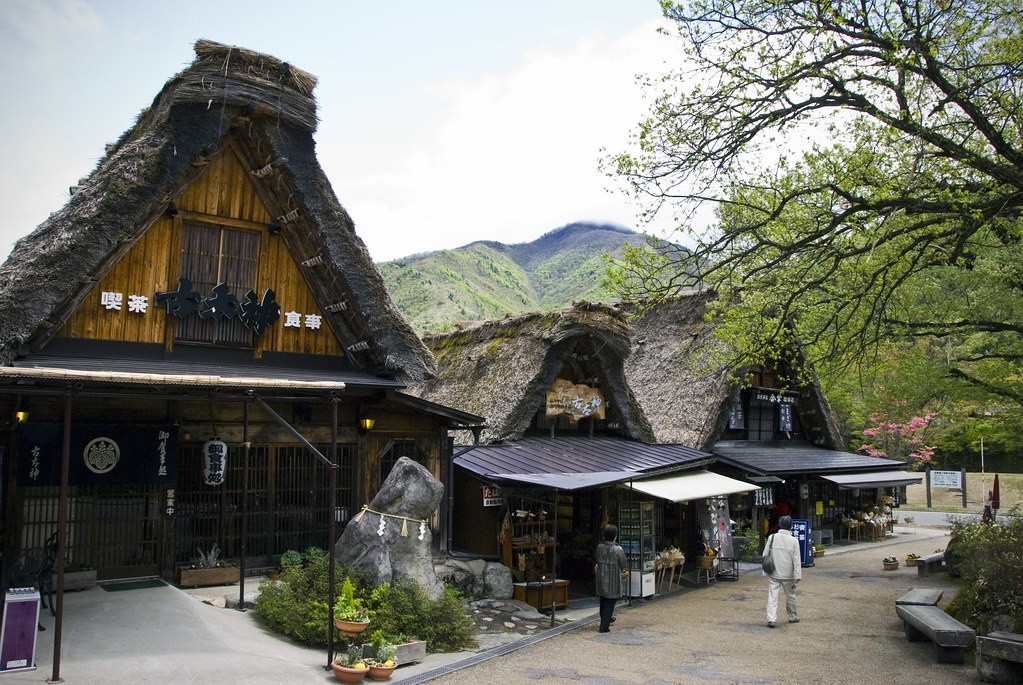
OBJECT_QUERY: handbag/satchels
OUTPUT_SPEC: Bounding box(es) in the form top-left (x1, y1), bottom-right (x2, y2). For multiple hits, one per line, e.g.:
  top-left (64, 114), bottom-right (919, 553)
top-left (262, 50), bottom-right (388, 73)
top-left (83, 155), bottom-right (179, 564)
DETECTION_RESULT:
top-left (761, 553), bottom-right (775, 574)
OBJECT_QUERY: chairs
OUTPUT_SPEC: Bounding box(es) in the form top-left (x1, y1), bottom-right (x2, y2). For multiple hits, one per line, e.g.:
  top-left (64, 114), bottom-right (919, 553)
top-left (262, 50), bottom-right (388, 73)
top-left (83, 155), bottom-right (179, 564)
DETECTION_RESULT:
top-left (3, 530), bottom-right (58, 631)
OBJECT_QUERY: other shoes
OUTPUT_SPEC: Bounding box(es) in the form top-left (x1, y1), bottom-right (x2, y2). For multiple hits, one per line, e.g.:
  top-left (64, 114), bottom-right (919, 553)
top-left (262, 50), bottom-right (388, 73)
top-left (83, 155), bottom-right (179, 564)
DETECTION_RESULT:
top-left (789, 618), bottom-right (800, 622)
top-left (599, 628), bottom-right (610, 632)
top-left (767, 621), bottom-right (776, 627)
top-left (610, 616), bottom-right (616, 623)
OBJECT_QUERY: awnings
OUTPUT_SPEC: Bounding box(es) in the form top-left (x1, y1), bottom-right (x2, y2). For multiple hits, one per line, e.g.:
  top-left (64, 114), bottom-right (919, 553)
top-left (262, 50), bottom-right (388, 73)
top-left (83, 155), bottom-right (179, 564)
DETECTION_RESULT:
top-left (617, 466), bottom-right (762, 505)
top-left (818, 470), bottom-right (923, 490)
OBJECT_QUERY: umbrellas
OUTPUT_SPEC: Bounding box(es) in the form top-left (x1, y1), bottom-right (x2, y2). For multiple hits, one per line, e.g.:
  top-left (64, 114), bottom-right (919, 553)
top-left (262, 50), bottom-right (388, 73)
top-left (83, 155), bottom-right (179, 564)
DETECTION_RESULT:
top-left (991, 473), bottom-right (1000, 521)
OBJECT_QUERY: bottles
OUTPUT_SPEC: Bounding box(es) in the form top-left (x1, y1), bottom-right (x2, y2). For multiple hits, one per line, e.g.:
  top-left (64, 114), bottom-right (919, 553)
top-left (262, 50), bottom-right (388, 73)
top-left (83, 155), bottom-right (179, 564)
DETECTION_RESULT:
top-left (620, 510), bottom-right (651, 570)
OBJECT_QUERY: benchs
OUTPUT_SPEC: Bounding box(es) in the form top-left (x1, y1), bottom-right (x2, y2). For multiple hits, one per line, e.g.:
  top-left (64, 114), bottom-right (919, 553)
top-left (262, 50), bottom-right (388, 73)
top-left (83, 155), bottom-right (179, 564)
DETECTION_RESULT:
top-left (895, 605), bottom-right (975, 664)
top-left (895, 588), bottom-right (943, 627)
top-left (915, 552), bottom-right (945, 577)
top-left (976, 630), bottom-right (1023, 684)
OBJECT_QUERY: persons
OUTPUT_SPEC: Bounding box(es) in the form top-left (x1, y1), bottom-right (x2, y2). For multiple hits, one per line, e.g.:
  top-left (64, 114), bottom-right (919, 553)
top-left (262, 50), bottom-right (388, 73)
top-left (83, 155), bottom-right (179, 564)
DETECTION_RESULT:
top-left (761, 516), bottom-right (802, 628)
top-left (594, 524), bottom-right (627, 632)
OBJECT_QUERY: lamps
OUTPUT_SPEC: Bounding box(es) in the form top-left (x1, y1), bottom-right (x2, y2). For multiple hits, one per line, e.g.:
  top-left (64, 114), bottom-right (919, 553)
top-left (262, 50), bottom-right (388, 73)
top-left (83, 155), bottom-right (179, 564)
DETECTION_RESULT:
top-left (356, 408), bottom-right (377, 436)
top-left (15, 401), bottom-right (31, 432)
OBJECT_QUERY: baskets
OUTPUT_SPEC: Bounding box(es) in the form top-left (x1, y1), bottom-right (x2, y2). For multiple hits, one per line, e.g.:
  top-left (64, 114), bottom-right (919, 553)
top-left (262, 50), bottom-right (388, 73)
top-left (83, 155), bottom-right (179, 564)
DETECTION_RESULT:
top-left (695, 556), bottom-right (717, 567)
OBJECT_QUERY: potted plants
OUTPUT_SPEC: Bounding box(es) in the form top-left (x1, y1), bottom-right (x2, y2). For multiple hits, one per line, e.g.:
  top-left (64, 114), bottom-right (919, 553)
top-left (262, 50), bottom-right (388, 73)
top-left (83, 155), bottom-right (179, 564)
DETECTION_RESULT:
top-left (330, 644), bottom-right (370, 685)
top-left (32, 561), bottom-right (98, 593)
top-left (905, 553), bottom-right (920, 566)
top-left (332, 576), bottom-right (370, 637)
top-left (177, 543), bottom-right (240, 588)
top-left (892, 514), bottom-right (899, 524)
top-left (904, 514), bottom-right (916, 523)
top-left (813, 543), bottom-right (826, 557)
top-left (364, 628), bottom-right (399, 681)
top-left (883, 556), bottom-right (899, 569)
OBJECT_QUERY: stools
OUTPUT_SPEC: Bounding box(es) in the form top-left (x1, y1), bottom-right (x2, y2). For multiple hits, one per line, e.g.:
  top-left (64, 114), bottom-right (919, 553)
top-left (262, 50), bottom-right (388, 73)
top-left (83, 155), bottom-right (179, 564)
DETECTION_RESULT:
top-left (696, 565), bottom-right (716, 584)
